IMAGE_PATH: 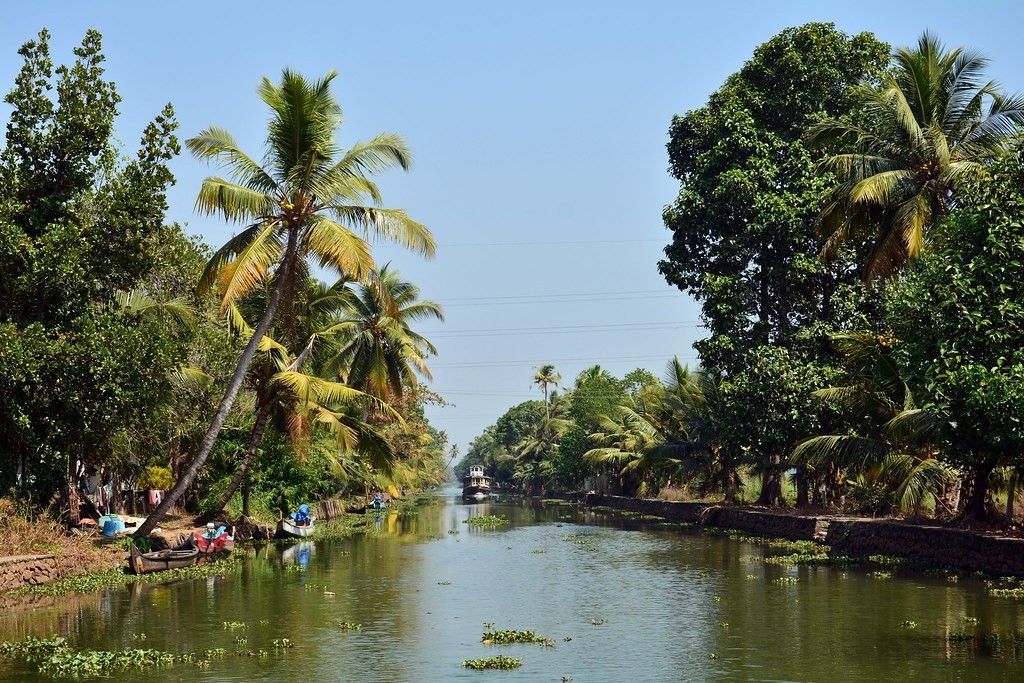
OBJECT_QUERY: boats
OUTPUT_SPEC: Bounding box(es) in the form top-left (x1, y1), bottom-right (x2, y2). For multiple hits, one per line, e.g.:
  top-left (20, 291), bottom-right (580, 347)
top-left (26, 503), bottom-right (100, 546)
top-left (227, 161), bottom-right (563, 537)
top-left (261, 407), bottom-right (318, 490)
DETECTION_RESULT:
top-left (189, 521), bottom-right (236, 556)
top-left (130, 530), bottom-right (202, 575)
top-left (461, 463), bottom-right (495, 503)
top-left (369, 497), bottom-right (387, 512)
top-left (275, 505), bottom-right (316, 536)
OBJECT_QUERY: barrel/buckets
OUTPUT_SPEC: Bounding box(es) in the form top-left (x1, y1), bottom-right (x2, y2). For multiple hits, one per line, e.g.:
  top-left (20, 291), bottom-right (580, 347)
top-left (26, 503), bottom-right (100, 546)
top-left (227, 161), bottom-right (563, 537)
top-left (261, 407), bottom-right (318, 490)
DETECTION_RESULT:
top-left (103, 521), bottom-right (116, 537)
